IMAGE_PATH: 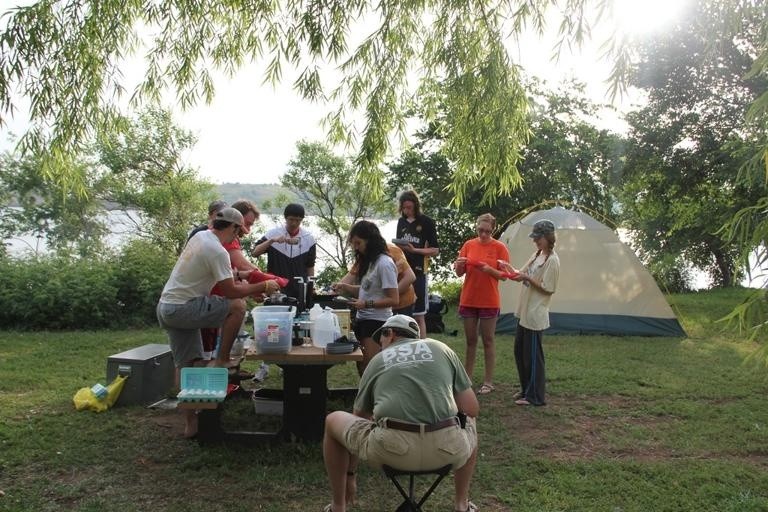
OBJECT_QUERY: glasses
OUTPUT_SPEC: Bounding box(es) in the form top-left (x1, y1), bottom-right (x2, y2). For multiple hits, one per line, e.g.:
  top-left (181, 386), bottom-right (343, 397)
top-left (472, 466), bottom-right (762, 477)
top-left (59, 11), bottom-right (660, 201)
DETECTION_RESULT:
top-left (476, 224), bottom-right (496, 235)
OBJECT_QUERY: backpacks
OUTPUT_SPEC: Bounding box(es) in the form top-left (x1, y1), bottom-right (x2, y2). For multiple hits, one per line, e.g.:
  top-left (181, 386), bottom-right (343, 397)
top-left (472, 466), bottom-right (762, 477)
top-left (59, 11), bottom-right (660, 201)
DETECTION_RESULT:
top-left (422, 294), bottom-right (450, 334)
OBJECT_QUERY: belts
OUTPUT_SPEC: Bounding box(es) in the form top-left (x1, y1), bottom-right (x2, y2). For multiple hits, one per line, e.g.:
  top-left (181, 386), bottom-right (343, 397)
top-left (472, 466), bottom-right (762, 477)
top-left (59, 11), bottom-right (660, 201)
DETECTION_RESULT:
top-left (376, 416), bottom-right (461, 434)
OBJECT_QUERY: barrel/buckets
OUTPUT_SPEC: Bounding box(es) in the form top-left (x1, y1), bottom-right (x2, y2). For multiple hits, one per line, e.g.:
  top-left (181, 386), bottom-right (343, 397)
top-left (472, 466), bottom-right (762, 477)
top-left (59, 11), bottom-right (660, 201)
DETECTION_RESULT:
top-left (314, 306), bottom-right (338, 348)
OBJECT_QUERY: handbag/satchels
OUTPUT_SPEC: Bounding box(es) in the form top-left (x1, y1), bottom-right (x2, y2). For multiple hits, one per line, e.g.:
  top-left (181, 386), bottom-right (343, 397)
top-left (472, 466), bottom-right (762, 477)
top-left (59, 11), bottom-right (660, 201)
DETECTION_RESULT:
top-left (72, 373), bottom-right (132, 413)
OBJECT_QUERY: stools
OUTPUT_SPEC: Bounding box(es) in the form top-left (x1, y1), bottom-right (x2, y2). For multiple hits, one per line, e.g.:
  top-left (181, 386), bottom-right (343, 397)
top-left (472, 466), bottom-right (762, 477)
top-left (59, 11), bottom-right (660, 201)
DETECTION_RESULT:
top-left (380, 459), bottom-right (454, 512)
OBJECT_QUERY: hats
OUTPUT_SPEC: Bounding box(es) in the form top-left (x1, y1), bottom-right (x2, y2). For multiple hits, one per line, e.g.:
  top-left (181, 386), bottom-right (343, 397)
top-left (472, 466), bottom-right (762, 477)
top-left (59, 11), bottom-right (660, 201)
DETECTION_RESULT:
top-left (215, 207), bottom-right (250, 234)
top-left (371, 314), bottom-right (421, 346)
top-left (208, 200), bottom-right (229, 214)
top-left (528, 220), bottom-right (556, 238)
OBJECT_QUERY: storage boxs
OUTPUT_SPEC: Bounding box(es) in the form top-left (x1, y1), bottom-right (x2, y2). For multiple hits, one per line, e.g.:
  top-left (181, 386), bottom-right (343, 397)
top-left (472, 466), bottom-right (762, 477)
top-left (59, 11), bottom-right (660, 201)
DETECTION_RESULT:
top-left (251, 385), bottom-right (283, 416)
top-left (249, 303), bottom-right (300, 355)
top-left (332, 308), bottom-right (351, 344)
top-left (105, 341), bottom-right (177, 406)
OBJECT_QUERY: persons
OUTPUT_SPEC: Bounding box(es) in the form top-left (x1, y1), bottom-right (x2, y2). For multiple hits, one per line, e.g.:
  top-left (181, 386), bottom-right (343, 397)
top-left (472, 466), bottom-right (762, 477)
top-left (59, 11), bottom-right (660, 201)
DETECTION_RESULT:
top-left (155, 207), bottom-right (279, 440)
top-left (192, 201), bottom-right (288, 365)
top-left (328, 220), bottom-right (399, 378)
top-left (337, 238), bottom-right (416, 317)
top-left (391, 189), bottom-right (440, 340)
top-left (181, 200), bottom-right (228, 242)
top-left (323, 314), bottom-right (483, 512)
top-left (251, 203), bottom-right (316, 383)
top-left (453, 212), bottom-right (511, 393)
top-left (500, 219), bottom-right (562, 408)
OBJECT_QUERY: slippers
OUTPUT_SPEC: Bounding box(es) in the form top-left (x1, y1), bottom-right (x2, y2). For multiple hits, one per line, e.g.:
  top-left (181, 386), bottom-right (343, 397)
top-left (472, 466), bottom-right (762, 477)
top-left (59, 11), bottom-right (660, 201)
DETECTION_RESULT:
top-left (209, 363), bottom-right (255, 382)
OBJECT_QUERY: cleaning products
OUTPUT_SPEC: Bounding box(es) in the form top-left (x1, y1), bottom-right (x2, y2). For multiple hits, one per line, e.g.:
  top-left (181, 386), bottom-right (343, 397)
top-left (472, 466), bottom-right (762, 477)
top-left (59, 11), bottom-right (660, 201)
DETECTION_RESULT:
top-left (310, 304), bottom-right (323, 338)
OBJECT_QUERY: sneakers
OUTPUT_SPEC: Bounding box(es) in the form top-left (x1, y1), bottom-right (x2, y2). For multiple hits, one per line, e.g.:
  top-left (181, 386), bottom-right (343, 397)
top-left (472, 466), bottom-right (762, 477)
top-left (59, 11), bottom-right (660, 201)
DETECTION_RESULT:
top-left (515, 398), bottom-right (532, 405)
top-left (512, 392), bottom-right (524, 399)
top-left (454, 499), bottom-right (480, 512)
top-left (324, 504), bottom-right (332, 512)
top-left (477, 383), bottom-right (496, 395)
top-left (252, 366), bottom-right (269, 383)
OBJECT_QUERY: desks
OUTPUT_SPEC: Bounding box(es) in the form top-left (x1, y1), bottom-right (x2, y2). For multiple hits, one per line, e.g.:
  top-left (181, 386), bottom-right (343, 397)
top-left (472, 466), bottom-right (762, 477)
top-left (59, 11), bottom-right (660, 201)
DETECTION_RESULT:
top-left (218, 327), bottom-right (367, 450)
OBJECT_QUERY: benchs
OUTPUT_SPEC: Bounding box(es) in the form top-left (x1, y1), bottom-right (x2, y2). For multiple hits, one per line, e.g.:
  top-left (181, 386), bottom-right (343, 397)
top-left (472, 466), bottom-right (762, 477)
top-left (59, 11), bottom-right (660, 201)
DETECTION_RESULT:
top-left (173, 350), bottom-right (247, 451)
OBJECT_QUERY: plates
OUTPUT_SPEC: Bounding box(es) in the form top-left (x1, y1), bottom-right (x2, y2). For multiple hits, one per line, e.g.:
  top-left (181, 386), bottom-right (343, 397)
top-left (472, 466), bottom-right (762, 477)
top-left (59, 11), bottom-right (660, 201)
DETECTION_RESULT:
top-left (310, 293), bottom-right (340, 300)
top-left (499, 271), bottom-right (520, 278)
top-left (392, 238), bottom-right (408, 245)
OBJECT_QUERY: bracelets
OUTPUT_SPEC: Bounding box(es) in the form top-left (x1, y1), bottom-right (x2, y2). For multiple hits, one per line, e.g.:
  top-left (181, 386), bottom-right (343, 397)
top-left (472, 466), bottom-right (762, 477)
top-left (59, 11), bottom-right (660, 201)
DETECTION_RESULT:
top-left (347, 471), bottom-right (356, 476)
top-left (264, 281), bottom-right (268, 291)
top-left (365, 299), bottom-right (375, 308)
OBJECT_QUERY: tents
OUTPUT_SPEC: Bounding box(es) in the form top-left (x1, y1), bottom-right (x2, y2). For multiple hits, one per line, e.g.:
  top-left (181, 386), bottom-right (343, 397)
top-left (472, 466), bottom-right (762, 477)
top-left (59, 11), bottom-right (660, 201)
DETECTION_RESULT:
top-left (479, 204), bottom-right (688, 339)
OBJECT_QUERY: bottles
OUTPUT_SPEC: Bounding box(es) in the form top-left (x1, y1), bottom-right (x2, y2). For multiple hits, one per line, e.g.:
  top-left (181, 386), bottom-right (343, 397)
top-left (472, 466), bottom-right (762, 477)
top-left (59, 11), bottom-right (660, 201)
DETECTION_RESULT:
top-left (308, 303), bottom-right (323, 340)
